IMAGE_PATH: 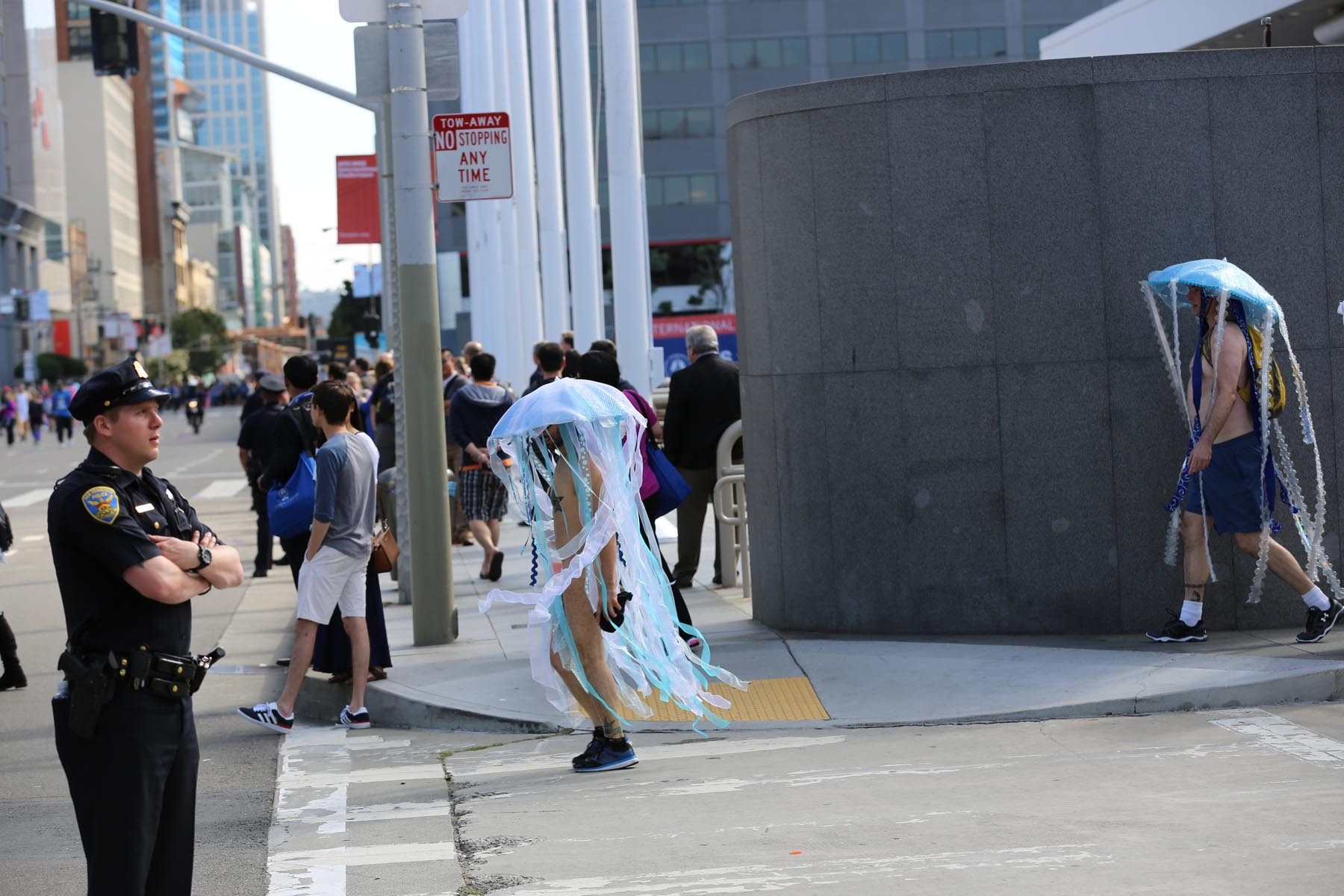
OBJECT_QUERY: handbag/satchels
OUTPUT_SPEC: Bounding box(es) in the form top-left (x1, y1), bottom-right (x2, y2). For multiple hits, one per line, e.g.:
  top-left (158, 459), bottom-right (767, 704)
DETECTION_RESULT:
top-left (266, 453), bottom-right (319, 538)
top-left (372, 527), bottom-right (401, 573)
top-left (628, 391), bottom-right (691, 517)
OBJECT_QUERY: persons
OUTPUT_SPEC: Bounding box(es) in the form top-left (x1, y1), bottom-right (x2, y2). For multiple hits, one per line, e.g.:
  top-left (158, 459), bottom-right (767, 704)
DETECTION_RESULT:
top-left (236, 322), bottom-right (743, 738)
top-left (0, 373), bottom-right (250, 447)
top-left (1141, 256), bottom-right (1344, 644)
top-left (45, 357), bottom-right (243, 896)
top-left (540, 380), bottom-right (640, 772)
top-left (0, 505), bottom-right (30, 692)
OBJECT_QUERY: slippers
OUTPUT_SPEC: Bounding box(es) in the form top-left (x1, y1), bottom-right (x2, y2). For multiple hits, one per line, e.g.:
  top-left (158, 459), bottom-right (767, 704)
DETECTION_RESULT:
top-left (480, 551), bottom-right (504, 582)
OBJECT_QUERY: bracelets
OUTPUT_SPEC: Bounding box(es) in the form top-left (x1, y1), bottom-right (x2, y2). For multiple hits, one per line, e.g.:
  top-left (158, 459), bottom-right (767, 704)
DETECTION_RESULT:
top-left (198, 579), bottom-right (212, 596)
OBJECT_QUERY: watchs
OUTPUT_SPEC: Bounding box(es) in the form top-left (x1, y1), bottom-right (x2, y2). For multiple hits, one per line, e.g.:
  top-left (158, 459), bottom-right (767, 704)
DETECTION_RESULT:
top-left (191, 543), bottom-right (212, 573)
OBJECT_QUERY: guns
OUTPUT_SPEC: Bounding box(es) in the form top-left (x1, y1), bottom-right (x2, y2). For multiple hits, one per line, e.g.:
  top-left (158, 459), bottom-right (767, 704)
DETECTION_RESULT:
top-left (57, 652), bottom-right (106, 740)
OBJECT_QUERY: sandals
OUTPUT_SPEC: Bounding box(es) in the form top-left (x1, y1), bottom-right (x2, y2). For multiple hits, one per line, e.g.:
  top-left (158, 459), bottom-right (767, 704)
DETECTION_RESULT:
top-left (328, 668), bottom-right (351, 683)
top-left (345, 668), bottom-right (387, 687)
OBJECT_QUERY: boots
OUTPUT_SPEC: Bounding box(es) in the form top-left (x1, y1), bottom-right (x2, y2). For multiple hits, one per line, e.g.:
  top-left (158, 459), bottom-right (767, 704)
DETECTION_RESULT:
top-left (0, 649), bottom-right (27, 691)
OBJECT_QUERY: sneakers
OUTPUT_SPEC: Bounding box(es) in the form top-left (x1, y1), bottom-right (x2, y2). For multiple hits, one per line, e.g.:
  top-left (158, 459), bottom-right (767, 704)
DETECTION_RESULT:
top-left (572, 729), bottom-right (639, 772)
top-left (335, 705), bottom-right (374, 729)
top-left (1296, 599), bottom-right (1344, 642)
top-left (1146, 607), bottom-right (1208, 642)
top-left (237, 702), bottom-right (295, 733)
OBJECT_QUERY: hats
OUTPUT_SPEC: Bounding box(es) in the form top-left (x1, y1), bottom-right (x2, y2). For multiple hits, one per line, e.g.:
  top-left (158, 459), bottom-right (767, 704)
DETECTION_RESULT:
top-left (259, 375), bottom-right (286, 393)
top-left (68, 358), bottom-right (171, 426)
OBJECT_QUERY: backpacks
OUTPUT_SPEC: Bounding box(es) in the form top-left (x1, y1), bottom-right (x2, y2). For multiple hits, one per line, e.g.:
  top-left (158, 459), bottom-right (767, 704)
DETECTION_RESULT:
top-left (1203, 321), bottom-right (1286, 419)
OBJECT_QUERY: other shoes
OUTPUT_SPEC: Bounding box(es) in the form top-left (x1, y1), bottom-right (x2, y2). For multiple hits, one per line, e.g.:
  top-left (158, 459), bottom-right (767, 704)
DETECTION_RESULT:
top-left (713, 577), bottom-right (722, 583)
top-left (686, 638), bottom-right (699, 647)
top-left (253, 568), bottom-right (268, 577)
top-left (463, 539), bottom-right (475, 546)
top-left (276, 657), bottom-right (291, 667)
top-left (672, 582), bottom-right (692, 588)
top-left (273, 553), bottom-right (290, 565)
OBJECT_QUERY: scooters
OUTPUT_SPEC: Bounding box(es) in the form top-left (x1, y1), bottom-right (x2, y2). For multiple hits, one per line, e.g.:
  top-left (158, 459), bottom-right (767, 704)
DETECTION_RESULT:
top-left (185, 394), bottom-right (203, 433)
